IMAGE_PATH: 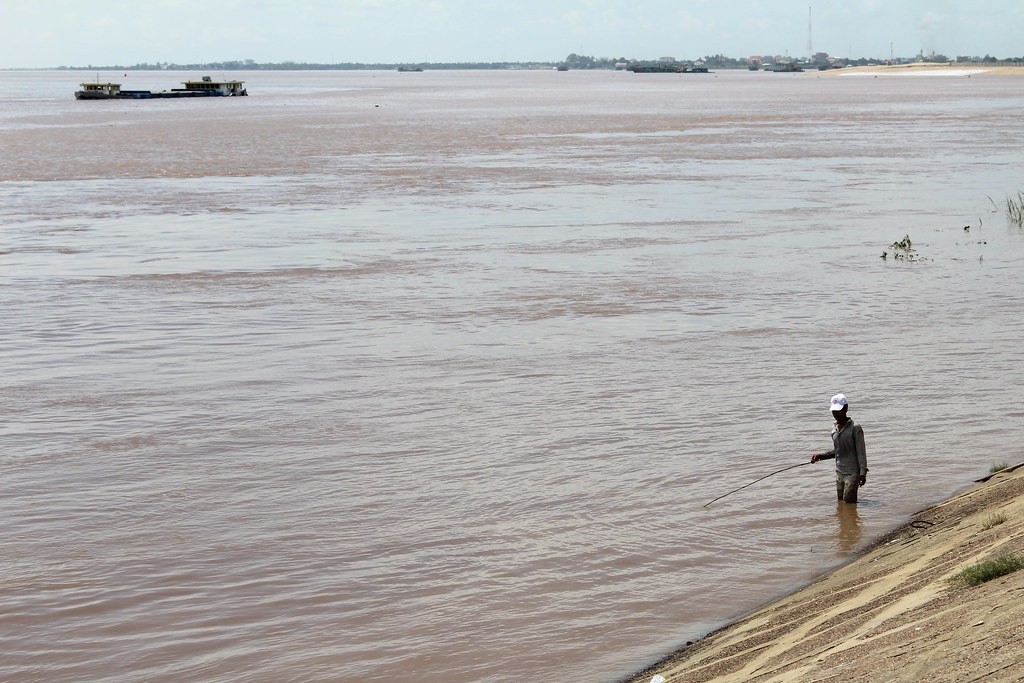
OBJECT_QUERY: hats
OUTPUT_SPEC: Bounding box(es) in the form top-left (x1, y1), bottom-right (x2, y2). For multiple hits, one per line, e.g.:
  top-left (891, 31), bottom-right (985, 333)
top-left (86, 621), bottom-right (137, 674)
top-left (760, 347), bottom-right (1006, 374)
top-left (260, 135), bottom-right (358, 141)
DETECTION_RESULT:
top-left (830, 394), bottom-right (848, 411)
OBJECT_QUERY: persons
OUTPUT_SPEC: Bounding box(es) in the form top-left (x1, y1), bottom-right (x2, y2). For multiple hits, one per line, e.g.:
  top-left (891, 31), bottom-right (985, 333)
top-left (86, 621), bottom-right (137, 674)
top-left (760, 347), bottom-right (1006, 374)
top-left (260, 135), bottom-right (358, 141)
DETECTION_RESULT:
top-left (811, 394), bottom-right (870, 503)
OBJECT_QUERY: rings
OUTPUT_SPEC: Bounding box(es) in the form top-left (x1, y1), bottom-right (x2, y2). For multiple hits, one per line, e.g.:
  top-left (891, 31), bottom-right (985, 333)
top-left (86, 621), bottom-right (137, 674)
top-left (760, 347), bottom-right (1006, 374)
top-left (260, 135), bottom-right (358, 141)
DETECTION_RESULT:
top-left (861, 481), bottom-right (862, 482)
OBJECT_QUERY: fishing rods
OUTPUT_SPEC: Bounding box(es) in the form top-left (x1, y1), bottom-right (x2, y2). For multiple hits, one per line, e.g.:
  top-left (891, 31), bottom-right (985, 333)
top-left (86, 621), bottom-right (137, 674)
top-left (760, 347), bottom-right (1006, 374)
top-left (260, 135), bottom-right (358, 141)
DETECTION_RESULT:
top-left (703, 459), bottom-right (816, 508)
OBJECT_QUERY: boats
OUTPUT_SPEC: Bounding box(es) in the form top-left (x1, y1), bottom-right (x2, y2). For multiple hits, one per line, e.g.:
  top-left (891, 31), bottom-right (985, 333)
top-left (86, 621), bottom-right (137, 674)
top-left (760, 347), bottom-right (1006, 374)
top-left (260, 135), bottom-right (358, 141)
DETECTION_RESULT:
top-left (74, 71), bottom-right (121, 100)
top-left (747, 56), bottom-right (806, 73)
top-left (397, 66), bottom-right (423, 72)
top-left (631, 61), bottom-right (709, 73)
top-left (180, 75), bottom-right (249, 97)
